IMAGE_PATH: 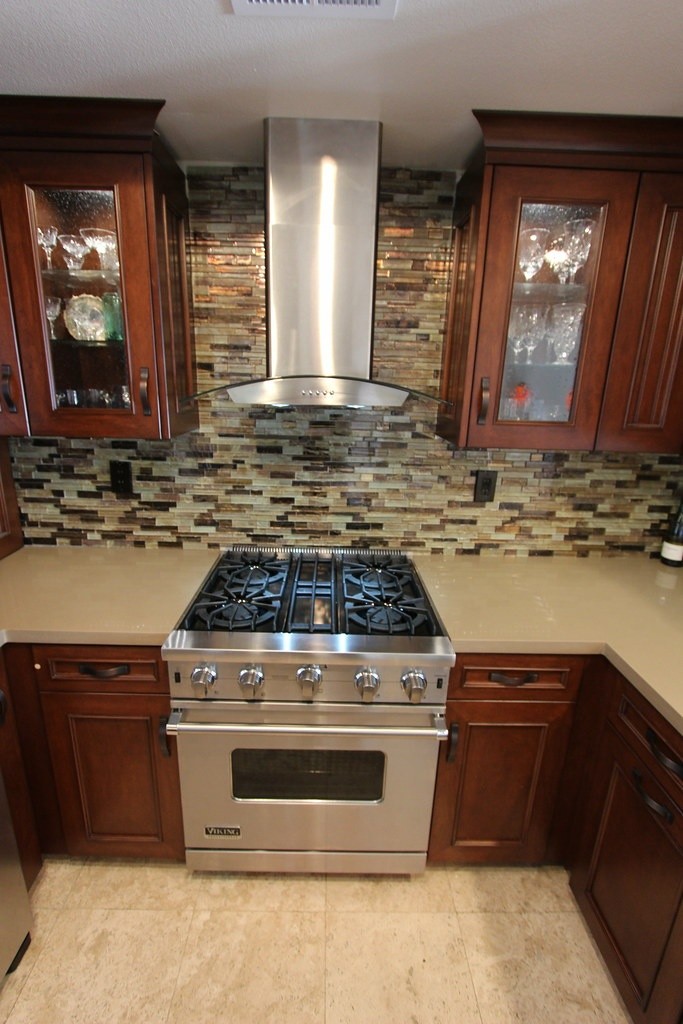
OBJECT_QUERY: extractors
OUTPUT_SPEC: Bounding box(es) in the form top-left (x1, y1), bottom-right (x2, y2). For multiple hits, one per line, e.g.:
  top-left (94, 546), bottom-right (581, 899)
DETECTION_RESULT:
top-left (180, 117), bottom-right (455, 412)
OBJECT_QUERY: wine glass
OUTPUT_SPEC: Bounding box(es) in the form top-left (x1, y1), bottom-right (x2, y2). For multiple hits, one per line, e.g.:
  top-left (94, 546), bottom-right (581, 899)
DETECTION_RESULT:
top-left (37, 224), bottom-right (124, 340)
top-left (508, 218), bottom-right (596, 366)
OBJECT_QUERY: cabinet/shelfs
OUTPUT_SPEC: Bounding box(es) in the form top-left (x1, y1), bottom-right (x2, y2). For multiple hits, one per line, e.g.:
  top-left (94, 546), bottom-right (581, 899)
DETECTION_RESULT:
top-left (432, 108), bottom-right (683, 455)
top-left (426, 652), bottom-right (581, 866)
top-left (1, 92), bottom-right (201, 438)
top-left (567, 670), bottom-right (683, 1024)
top-left (5, 645), bottom-right (186, 862)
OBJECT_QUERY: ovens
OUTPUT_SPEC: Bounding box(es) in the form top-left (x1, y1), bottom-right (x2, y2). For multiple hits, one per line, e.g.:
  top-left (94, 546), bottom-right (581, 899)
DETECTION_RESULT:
top-left (166, 708), bottom-right (450, 878)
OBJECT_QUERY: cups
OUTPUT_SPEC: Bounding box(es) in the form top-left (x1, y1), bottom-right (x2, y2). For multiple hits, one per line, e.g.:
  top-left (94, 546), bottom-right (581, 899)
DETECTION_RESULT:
top-left (498, 399), bottom-right (569, 421)
top-left (56, 385), bottom-right (130, 410)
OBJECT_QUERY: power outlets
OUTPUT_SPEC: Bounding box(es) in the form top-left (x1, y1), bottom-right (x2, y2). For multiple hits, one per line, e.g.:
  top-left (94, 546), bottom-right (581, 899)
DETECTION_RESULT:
top-left (474, 470), bottom-right (498, 502)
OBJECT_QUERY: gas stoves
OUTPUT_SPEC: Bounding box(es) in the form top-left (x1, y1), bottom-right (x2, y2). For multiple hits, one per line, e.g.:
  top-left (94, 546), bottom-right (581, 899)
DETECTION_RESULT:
top-left (160, 547), bottom-right (455, 716)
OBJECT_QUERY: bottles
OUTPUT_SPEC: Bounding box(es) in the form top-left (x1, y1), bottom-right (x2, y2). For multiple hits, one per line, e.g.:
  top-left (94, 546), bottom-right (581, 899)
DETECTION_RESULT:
top-left (660, 494), bottom-right (683, 568)
